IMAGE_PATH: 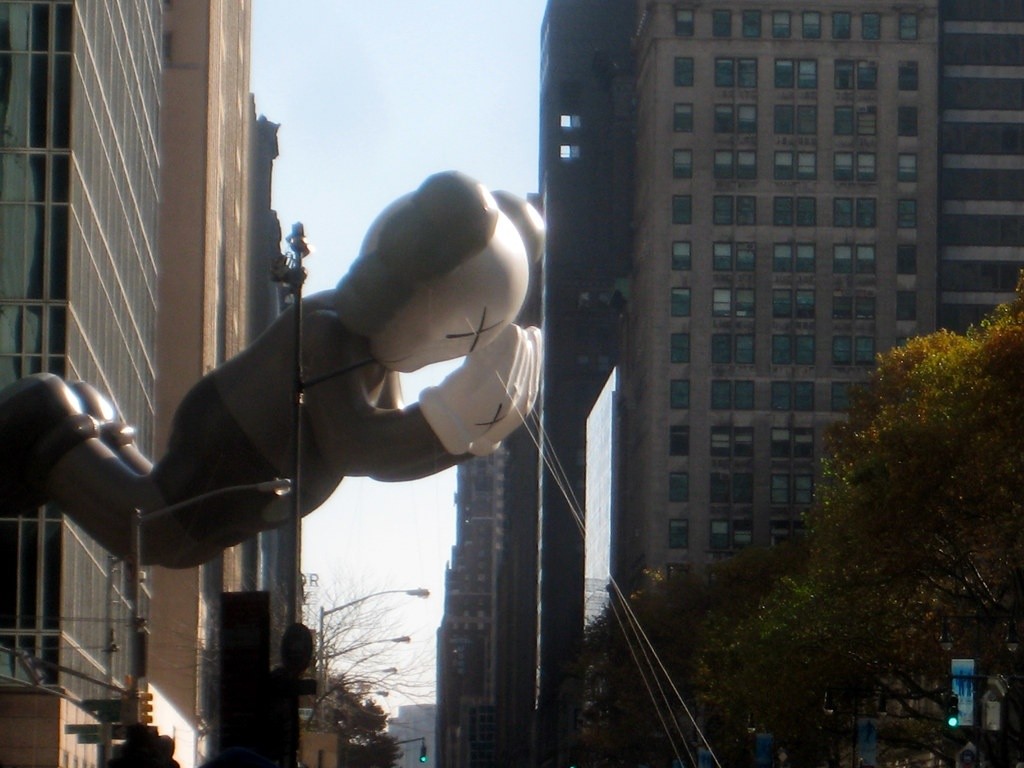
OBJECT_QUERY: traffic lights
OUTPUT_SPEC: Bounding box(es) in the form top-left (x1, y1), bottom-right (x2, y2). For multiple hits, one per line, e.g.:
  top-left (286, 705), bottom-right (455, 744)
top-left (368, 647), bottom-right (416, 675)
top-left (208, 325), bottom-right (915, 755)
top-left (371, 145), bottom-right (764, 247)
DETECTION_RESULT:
top-left (946, 691), bottom-right (961, 732)
top-left (137, 689), bottom-right (154, 728)
top-left (420, 745), bottom-right (427, 762)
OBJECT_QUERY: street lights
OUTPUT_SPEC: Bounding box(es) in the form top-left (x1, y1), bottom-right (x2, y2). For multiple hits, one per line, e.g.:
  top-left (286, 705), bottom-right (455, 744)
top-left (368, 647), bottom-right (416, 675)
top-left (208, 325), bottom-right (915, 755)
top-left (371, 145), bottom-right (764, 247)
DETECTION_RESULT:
top-left (315, 589), bottom-right (430, 728)
top-left (124, 478), bottom-right (292, 735)
